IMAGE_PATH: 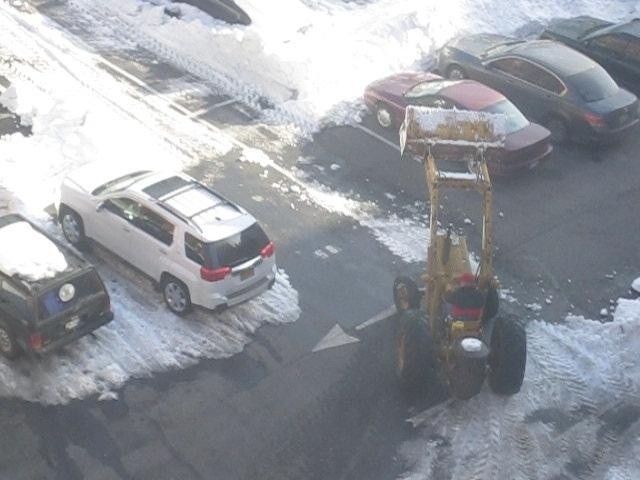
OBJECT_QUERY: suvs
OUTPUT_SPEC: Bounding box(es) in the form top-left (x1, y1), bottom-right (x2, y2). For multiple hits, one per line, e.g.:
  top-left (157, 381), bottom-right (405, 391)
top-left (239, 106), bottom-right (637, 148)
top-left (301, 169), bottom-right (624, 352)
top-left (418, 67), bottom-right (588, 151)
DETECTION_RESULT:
top-left (0, 210), bottom-right (114, 361)
top-left (58, 160), bottom-right (276, 315)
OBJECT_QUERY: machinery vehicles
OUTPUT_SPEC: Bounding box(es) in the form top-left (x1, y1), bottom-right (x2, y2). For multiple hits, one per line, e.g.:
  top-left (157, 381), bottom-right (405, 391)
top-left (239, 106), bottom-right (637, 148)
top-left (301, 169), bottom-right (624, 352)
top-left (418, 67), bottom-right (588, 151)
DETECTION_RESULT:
top-left (395, 104), bottom-right (526, 401)
